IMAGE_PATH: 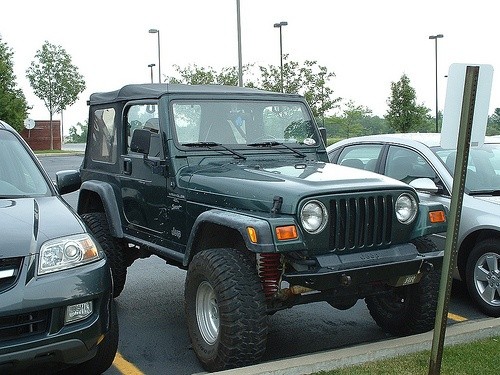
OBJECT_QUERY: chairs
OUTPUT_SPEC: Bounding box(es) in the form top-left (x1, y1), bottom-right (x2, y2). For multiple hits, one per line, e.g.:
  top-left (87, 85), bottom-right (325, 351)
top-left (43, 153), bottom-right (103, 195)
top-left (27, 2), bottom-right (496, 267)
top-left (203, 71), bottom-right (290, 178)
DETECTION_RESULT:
top-left (341, 158), bottom-right (378, 172)
top-left (142, 118), bottom-right (161, 157)
top-left (198, 117), bottom-right (239, 144)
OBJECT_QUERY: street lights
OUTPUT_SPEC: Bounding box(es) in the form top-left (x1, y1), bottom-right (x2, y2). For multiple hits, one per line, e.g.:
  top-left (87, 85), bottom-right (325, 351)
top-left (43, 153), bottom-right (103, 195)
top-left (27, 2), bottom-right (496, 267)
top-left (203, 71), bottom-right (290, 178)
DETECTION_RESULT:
top-left (148, 29), bottom-right (160, 85)
top-left (274, 21), bottom-right (288, 94)
top-left (148, 63), bottom-right (155, 84)
top-left (428, 34), bottom-right (444, 133)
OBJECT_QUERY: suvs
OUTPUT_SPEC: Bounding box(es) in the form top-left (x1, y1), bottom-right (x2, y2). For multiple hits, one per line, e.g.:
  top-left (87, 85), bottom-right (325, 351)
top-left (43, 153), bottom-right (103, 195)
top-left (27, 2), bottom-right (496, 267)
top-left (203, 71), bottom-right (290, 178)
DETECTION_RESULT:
top-left (75, 83), bottom-right (450, 373)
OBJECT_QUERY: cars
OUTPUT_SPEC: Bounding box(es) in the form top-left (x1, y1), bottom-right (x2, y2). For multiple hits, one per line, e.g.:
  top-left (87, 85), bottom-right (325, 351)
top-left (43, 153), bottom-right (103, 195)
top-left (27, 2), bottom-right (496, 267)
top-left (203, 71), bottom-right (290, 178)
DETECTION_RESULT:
top-left (326, 132), bottom-right (500, 319)
top-left (0, 121), bottom-right (120, 375)
